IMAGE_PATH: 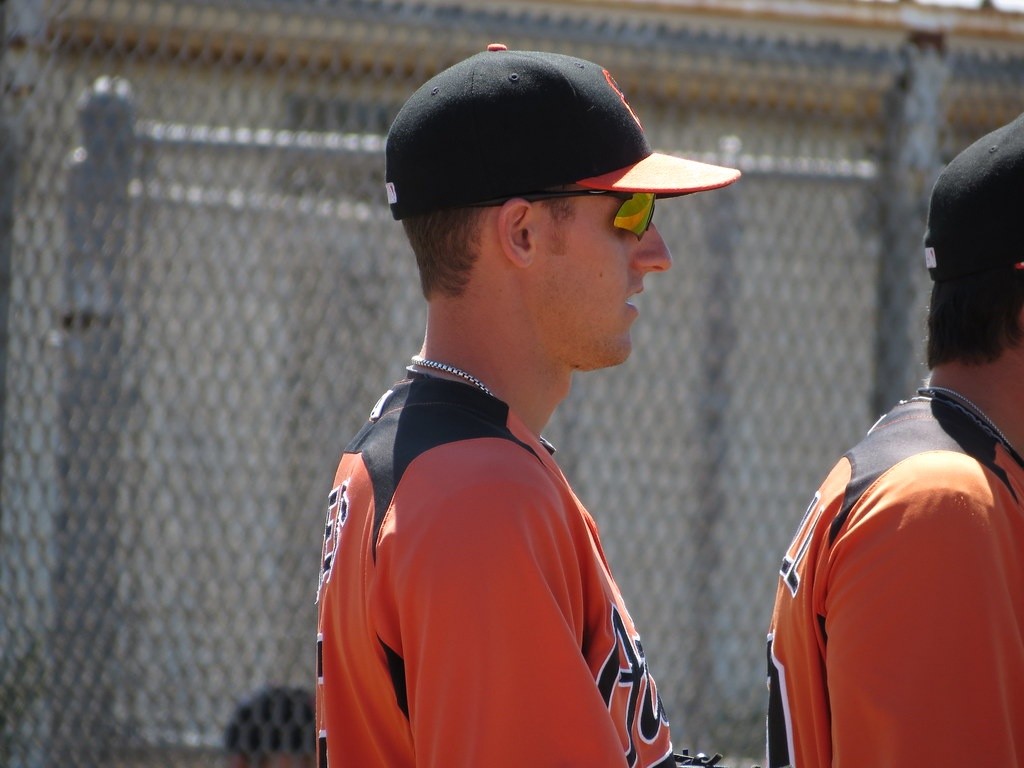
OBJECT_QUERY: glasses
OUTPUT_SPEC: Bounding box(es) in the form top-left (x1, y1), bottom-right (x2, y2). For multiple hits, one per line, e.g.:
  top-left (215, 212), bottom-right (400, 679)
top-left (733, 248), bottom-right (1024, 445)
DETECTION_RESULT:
top-left (463, 189), bottom-right (656, 242)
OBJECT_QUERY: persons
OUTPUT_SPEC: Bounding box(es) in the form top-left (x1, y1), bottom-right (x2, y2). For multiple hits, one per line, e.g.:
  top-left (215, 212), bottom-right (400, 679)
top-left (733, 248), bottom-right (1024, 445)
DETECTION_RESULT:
top-left (314, 41), bottom-right (725, 768)
top-left (764, 108), bottom-right (1024, 768)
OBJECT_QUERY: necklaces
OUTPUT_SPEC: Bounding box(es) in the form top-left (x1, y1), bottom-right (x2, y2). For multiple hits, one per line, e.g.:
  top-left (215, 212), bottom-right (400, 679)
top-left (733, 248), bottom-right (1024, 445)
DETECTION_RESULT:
top-left (411, 354), bottom-right (557, 456)
top-left (916, 386), bottom-right (1024, 461)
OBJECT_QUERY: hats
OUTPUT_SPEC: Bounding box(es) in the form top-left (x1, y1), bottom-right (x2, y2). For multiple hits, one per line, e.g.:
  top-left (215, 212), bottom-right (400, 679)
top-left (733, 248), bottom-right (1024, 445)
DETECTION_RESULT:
top-left (923, 112), bottom-right (1024, 282)
top-left (386, 43), bottom-right (741, 223)
